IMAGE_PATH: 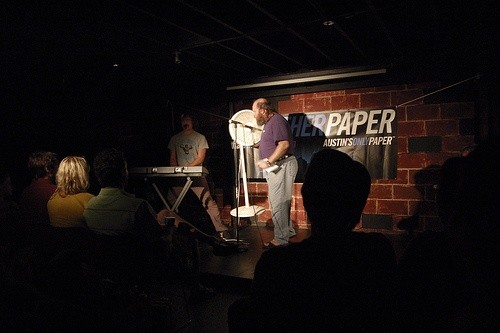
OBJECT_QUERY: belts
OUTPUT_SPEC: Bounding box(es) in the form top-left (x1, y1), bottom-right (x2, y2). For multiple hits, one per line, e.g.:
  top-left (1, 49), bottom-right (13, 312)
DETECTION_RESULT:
top-left (276, 153), bottom-right (292, 161)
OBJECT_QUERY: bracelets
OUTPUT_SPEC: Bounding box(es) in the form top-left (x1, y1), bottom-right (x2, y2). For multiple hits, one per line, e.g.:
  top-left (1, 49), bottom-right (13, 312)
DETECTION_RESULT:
top-left (266, 160), bottom-right (272, 167)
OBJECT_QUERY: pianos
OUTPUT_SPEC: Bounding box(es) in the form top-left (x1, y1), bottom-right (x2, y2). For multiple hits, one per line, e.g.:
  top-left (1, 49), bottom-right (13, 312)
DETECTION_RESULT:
top-left (135, 165), bottom-right (211, 178)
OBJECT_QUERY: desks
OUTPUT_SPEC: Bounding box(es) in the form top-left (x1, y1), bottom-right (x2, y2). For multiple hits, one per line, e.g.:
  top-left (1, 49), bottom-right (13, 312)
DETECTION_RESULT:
top-left (140, 173), bottom-right (203, 216)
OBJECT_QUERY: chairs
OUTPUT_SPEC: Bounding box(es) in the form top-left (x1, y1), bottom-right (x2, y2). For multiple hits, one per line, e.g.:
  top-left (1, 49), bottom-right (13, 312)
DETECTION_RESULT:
top-left (44, 224), bottom-right (193, 333)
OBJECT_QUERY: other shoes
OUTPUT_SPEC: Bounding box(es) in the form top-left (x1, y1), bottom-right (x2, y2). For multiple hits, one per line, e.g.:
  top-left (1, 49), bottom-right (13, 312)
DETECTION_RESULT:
top-left (263, 241), bottom-right (289, 248)
top-left (219, 231), bottom-right (242, 241)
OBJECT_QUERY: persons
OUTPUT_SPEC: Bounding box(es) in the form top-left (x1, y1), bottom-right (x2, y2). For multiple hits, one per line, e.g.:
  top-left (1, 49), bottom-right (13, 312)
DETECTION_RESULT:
top-left (398, 154), bottom-right (500, 332)
top-left (82, 146), bottom-right (217, 303)
top-left (47, 155), bottom-right (97, 227)
top-left (164, 112), bottom-right (233, 239)
top-left (226, 147), bottom-right (398, 333)
top-left (0, 167), bottom-right (20, 228)
top-left (250, 97), bottom-right (298, 250)
top-left (19, 151), bottom-right (59, 226)
